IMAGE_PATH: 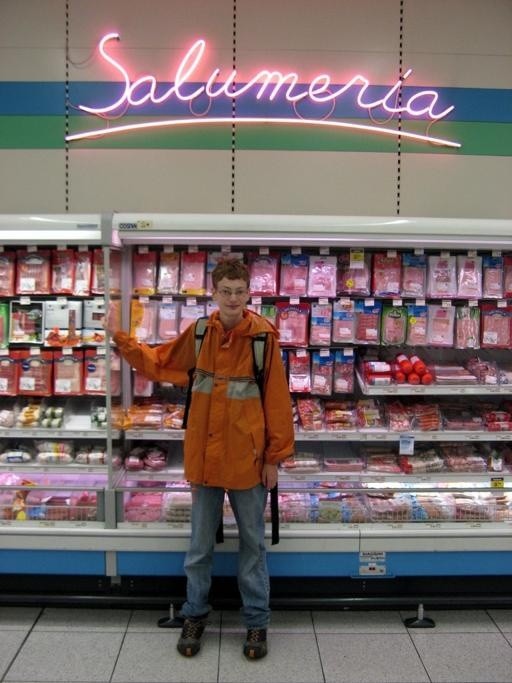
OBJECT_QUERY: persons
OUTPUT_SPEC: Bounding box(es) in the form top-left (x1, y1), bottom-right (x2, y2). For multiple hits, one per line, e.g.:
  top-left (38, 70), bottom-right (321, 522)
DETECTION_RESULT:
top-left (98, 256), bottom-right (299, 660)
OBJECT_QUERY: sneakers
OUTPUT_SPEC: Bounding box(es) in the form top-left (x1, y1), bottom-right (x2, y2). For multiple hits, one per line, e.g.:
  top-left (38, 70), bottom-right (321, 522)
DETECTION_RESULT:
top-left (244, 628), bottom-right (267, 659)
top-left (177, 617), bottom-right (206, 657)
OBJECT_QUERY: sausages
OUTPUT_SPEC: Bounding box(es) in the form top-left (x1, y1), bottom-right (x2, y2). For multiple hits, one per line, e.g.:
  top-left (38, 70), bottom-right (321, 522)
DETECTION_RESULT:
top-left (392, 354), bottom-right (434, 385)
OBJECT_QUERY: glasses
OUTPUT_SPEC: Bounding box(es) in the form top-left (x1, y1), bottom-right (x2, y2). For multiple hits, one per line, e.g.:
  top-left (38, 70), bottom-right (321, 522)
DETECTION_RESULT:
top-left (216, 287), bottom-right (249, 297)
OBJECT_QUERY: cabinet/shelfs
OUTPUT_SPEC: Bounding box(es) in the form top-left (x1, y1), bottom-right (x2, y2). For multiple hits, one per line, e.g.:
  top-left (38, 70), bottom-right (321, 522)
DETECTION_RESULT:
top-left (0, 213), bottom-right (512, 629)
